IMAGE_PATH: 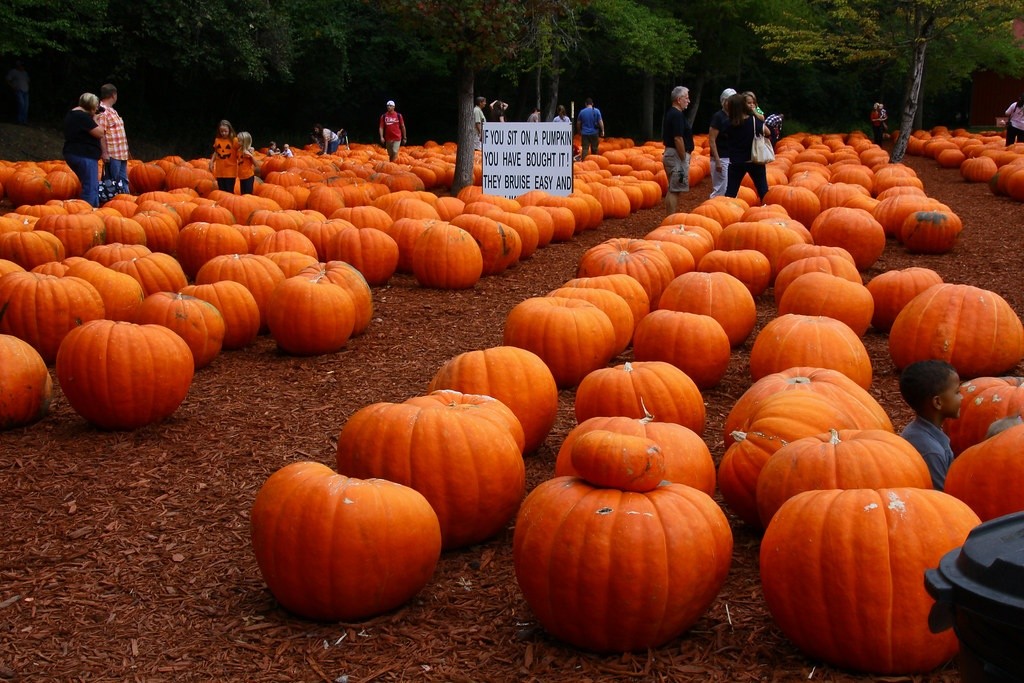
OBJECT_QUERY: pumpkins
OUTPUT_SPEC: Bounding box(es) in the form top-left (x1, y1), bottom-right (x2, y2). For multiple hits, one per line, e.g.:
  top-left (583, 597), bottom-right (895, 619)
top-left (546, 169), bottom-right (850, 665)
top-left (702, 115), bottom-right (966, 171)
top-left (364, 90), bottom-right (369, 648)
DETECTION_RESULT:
top-left (0, 133), bottom-right (962, 430)
top-left (513, 475), bottom-right (735, 651)
top-left (939, 376), bottom-right (1024, 522)
top-left (890, 126), bottom-right (1024, 201)
top-left (249, 461), bottom-right (442, 619)
top-left (717, 391), bottom-right (861, 531)
top-left (887, 283), bottom-right (1024, 380)
top-left (556, 396), bottom-right (716, 497)
top-left (757, 487), bottom-right (985, 672)
top-left (756, 427), bottom-right (934, 529)
top-left (337, 400), bottom-right (526, 542)
top-left (569, 430), bottom-right (667, 492)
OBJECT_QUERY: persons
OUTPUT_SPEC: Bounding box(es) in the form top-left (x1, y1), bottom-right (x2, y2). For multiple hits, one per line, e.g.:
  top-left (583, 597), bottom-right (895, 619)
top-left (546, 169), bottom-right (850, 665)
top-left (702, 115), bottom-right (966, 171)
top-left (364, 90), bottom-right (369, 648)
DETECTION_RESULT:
top-left (471, 97), bottom-right (487, 152)
top-left (870, 103), bottom-right (888, 147)
top-left (553, 105), bottom-right (571, 122)
top-left (267, 142), bottom-right (294, 159)
top-left (1005, 95), bottom-right (1024, 147)
top-left (92, 84), bottom-right (130, 203)
top-left (6, 58), bottom-right (31, 126)
top-left (899, 359), bottom-right (963, 492)
top-left (236, 132), bottom-right (257, 195)
top-left (62, 93), bottom-right (104, 207)
top-left (577, 97), bottom-right (605, 161)
top-left (527, 108), bottom-right (543, 122)
top-left (662, 86), bottom-right (694, 216)
top-left (209, 120), bottom-right (255, 194)
top-left (379, 100), bottom-right (407, 162)
top-left (709, 88), bottom-right (785, 201)
top-left (314, 124), bottom-right (339, 156)
top-left (310, 128), bottom-right (349, 148)
top-left (490, 100), bottom-right (509, 122)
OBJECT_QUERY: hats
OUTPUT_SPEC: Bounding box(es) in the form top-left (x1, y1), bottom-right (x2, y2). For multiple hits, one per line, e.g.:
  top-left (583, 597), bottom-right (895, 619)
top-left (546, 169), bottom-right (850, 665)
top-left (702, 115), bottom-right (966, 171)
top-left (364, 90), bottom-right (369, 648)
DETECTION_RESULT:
top-left (874, 103), bottom-right (883, 109)
top-left (387, 101), bottom-right (395, 106)
top-left (721, 88), bottom-right (736, 105)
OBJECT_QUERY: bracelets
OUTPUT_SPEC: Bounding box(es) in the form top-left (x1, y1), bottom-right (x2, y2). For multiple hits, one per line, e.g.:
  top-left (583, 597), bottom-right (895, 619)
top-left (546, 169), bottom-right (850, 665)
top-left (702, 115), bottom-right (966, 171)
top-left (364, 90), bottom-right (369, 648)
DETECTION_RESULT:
top-left (324, 151), bottom-right (327, 152)
top-left (403, 138), bottom-right (407, 140)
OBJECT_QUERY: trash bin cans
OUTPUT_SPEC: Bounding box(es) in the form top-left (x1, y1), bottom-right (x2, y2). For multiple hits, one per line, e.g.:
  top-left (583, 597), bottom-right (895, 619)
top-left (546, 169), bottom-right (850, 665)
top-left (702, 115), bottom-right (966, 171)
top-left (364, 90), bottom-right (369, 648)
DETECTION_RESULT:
top-left (923, 509), bottom-right (1024, 683)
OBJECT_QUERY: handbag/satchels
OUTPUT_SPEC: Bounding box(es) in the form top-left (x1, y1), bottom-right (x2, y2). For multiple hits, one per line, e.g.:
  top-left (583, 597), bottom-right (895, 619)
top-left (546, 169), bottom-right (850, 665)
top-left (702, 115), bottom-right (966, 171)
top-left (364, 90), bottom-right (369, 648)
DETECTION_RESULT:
top-left (750, 116), bottom-right (775, 164)
top-left (97, 160), bottom-right (125, 203)
